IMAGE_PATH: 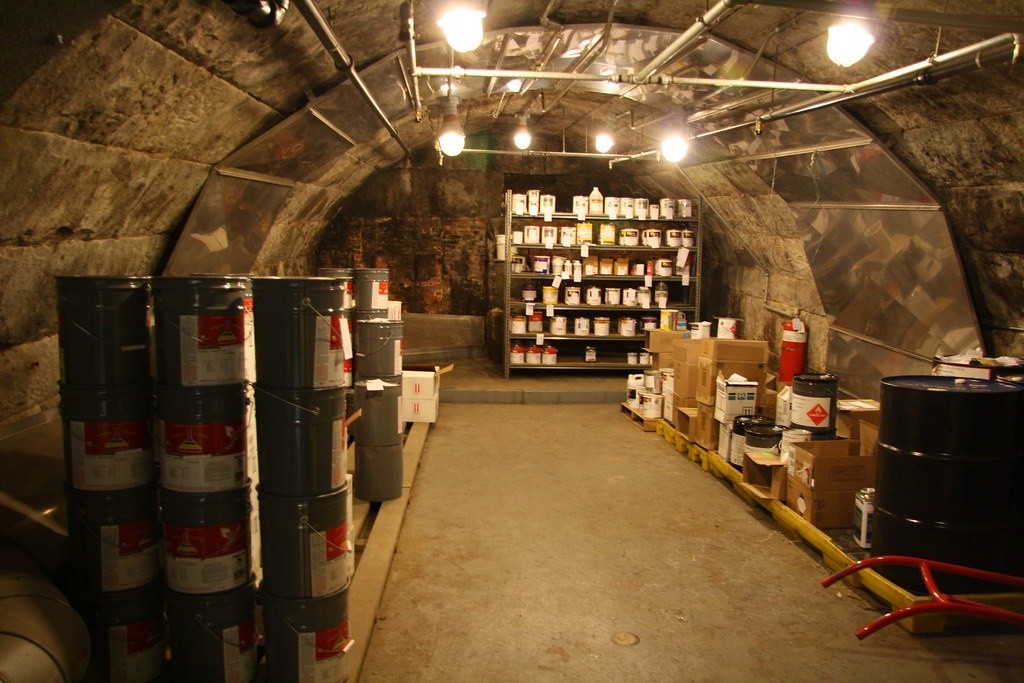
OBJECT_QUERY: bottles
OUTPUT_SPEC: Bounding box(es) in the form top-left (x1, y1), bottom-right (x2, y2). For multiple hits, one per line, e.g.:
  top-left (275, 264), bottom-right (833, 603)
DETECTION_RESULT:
top-left (589, 187), bottom-right (604, 216)
top-left (515, 261), bottom-right (521, 273)
top-left (646, 257), bottom-right (654, 276)
top-left (627, 374), bottom-right (643, 407)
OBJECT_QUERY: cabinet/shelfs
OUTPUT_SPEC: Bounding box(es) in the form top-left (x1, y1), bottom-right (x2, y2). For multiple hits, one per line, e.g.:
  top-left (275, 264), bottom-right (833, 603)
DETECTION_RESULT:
top-left (503, 189), bottom-right (702, 380)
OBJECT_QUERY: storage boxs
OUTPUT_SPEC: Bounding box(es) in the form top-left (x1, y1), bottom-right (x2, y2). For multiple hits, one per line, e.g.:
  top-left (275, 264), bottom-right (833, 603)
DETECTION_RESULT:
top-left (614, 256), bottom-right (629, 276)
top-left (641, 326), bottom-right (886, 528)
top-left (582, 256), bottom-right (598, 276)
top-left (402, 362), bottom-right (455, 400)
top-left (599, 258), bottom-right (613, 274)
top-left (401, 395), bottom-right (439, 423)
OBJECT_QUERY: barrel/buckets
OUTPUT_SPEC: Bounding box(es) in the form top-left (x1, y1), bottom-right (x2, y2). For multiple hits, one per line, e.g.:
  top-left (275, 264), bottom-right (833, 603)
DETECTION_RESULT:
top-left (728, 415), bottom-right (774, 472)
top-left (779, 428), bottom-right (811, 466)
top-left (717, 317), bottom-right (737, 339)
top-left (494, 234), bottom-right (518, 260)
top-left (355, 267), bottom-right (404, 502)
top-left (793, 373), bottom-right (840, 443)
top-left (870, 356), bottom-right (1024, 594)
top-left (691, 321), bottom-right (711, 339)
top-left (353, 309), bottom-right (388, 363)
top-left (59, 267), bottom-right (352, 683)
top-left (509, 189), bottom-right (694, 364)
top-left (744, 425), bottom-right (787, 487)
top-left (638, 368), bottom-right (674, 420)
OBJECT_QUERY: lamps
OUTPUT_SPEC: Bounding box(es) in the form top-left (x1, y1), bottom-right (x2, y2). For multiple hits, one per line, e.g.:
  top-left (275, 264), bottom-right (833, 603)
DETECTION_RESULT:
top-left (437, 96), bottom-right (467, 157)
top-left (514, 112), bottom-right (531, 150)
